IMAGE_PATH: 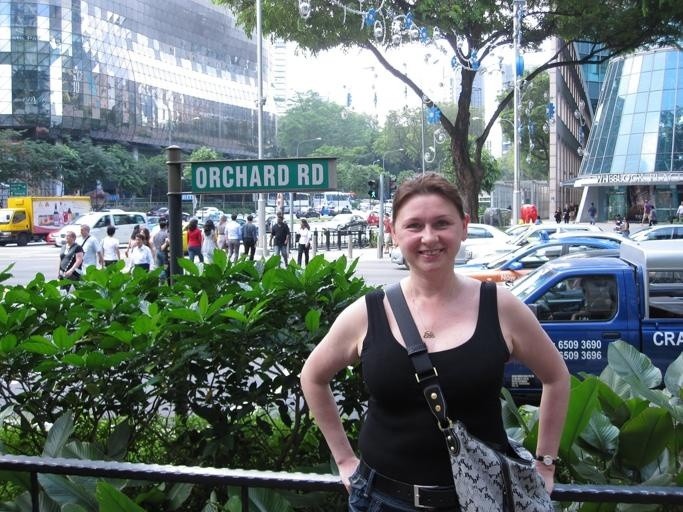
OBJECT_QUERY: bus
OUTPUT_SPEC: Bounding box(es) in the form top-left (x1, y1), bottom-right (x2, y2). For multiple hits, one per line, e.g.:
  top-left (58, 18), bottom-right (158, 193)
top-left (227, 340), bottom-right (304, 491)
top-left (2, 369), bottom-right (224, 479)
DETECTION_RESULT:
top-left (266, 191), bottom-right (311, 217)
top-left (313, 191), bottom-right (357, 213)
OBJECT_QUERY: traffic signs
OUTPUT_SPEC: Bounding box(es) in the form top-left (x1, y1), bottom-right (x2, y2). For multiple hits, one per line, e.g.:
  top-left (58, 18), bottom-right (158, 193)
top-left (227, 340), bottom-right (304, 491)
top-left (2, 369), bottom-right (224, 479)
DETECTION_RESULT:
top-left (7, 181), bottom-right (29, 198)
top-left (186, 155), bottom-right (338, 194)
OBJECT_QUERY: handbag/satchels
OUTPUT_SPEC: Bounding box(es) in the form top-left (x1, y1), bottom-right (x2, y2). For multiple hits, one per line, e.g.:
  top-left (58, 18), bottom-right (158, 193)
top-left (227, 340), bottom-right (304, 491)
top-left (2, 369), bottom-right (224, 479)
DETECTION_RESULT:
top-left (437, 419), bottom-right (556, 512)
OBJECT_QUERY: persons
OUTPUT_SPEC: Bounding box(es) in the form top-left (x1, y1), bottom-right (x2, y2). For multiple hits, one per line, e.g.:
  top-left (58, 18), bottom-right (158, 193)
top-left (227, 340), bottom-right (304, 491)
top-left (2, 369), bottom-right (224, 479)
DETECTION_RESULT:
top-left (382, 213), bottom-right (392, 254)
top-left (534, 199), bottom-right (683, 237)
top-left (301, 173), bottom-right (571, 511)
top-left (548, 279), bottom-right (611, 321)
top-left (58, 215), bottom-right (312, 292)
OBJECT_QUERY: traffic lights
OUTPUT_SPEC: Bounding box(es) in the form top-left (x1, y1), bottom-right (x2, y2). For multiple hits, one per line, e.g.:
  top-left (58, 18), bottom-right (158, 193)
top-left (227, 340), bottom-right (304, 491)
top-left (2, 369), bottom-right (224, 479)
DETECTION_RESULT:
top-left (366, 179), bottom-right (377, 200)
top-left (387, 177), bottom-right (398, 201)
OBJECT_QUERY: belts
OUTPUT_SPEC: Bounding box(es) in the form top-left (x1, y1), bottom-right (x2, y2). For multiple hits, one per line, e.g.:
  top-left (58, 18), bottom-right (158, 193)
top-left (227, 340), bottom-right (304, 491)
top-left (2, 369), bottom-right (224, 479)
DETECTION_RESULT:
top-left (358, 454), bottom-right (459, 510)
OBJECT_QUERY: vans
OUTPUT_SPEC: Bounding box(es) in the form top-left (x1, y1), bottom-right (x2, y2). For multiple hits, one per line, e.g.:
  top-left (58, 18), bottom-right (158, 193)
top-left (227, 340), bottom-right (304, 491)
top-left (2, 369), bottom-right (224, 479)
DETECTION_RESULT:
top-left (56, 212), bottom-right (151, 254)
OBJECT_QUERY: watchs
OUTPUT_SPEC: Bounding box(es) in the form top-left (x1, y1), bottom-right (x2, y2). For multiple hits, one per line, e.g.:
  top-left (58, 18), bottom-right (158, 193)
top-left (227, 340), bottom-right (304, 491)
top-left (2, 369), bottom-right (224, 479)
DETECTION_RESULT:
top-left (535, 455), bottom-right (559, 466)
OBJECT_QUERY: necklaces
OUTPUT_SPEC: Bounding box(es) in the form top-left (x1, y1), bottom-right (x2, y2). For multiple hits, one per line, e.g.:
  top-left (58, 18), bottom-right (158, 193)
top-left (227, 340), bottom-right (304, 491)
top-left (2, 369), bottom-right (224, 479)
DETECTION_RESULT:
top-left (411, 285), bottom-right (452, 339)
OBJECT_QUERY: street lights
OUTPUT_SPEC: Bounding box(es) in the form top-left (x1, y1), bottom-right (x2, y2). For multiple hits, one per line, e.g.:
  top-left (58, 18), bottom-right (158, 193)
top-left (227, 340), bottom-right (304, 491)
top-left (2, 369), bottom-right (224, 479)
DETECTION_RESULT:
top-left (371, 158), bottom-right (380, 167)
top-left (295, 138), bottom-right (325, 157)
top-left (471, 114), bottom-right (521, 222)
top-left (381, 148), bottom-right (407, 175)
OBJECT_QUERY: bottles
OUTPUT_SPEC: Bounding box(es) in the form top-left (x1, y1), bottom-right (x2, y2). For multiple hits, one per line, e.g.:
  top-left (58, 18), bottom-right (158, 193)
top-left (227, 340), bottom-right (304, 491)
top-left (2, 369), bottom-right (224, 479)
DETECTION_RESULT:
top-left (53, 203), bottom-right (60, 227)
top-left (59, 202), bottom-right (72, 228)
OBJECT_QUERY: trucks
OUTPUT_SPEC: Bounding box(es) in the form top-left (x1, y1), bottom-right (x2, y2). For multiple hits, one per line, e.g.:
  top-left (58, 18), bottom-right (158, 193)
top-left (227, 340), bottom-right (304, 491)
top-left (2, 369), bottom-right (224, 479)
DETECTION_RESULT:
top-left (0, 195), bottom-right (95, 248)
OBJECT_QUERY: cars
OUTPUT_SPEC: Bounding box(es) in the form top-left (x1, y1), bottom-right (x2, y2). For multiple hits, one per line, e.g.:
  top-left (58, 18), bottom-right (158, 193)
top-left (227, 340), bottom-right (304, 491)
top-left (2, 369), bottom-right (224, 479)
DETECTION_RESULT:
top-left (625, 203), bottom-right (678, 222)
top-left (45, 214), bottom-right (89, 247)
top-left (101, 209), bottom-right (125, 212)
top-left (142, 200), bottom-right (393, 259)
